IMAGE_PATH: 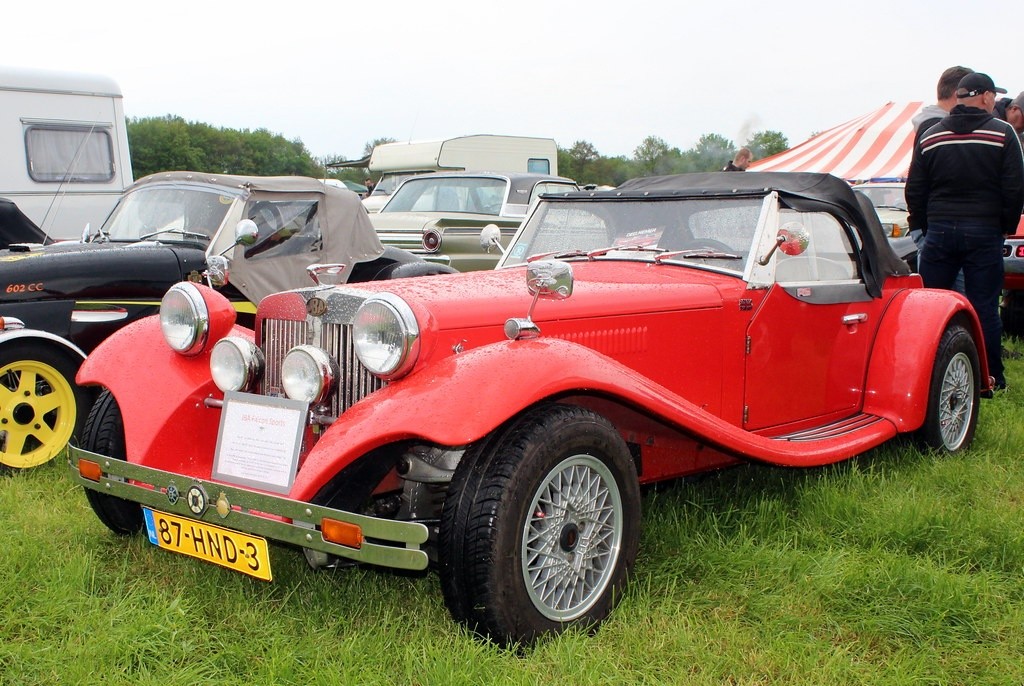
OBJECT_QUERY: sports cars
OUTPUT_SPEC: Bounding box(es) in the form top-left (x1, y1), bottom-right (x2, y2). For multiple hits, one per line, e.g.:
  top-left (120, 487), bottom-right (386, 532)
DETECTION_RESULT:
top-left (0, 159), bottom-right (464, 477)
top-left (65, 171), bottom-right (1000, 660)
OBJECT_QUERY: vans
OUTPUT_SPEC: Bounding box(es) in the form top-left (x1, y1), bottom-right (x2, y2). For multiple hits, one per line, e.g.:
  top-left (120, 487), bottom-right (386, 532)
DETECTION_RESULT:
top-left (0, 69), bottom-right (626, 290)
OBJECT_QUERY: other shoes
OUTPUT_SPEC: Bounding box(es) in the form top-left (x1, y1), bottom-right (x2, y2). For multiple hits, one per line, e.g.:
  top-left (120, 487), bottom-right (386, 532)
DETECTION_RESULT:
top-left (993, 378), bottom-right (1006, 390)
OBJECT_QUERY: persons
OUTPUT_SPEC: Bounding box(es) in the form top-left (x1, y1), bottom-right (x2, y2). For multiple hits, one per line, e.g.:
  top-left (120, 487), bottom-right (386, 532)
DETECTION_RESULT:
top-left (723, 149), bottom-right (752, 172)
top-left (905, 65), bottom-right (1024, 393)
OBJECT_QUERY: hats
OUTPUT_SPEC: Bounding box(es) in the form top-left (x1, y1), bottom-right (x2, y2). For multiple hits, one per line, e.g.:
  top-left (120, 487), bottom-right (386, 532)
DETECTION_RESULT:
top-left (956, 72), bottom-right (1007, 98)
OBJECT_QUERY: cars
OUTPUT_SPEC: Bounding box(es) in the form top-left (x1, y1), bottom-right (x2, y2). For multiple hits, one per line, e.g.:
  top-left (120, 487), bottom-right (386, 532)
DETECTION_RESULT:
top-left (1000, 213), bottom-right (1024, 334)
top-left (808, 177), bottom-right (922, 278)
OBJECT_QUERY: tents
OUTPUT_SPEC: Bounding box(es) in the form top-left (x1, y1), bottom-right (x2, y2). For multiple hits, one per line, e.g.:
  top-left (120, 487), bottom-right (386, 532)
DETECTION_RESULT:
top-left (724, 101), bottom-right (938, 206)
